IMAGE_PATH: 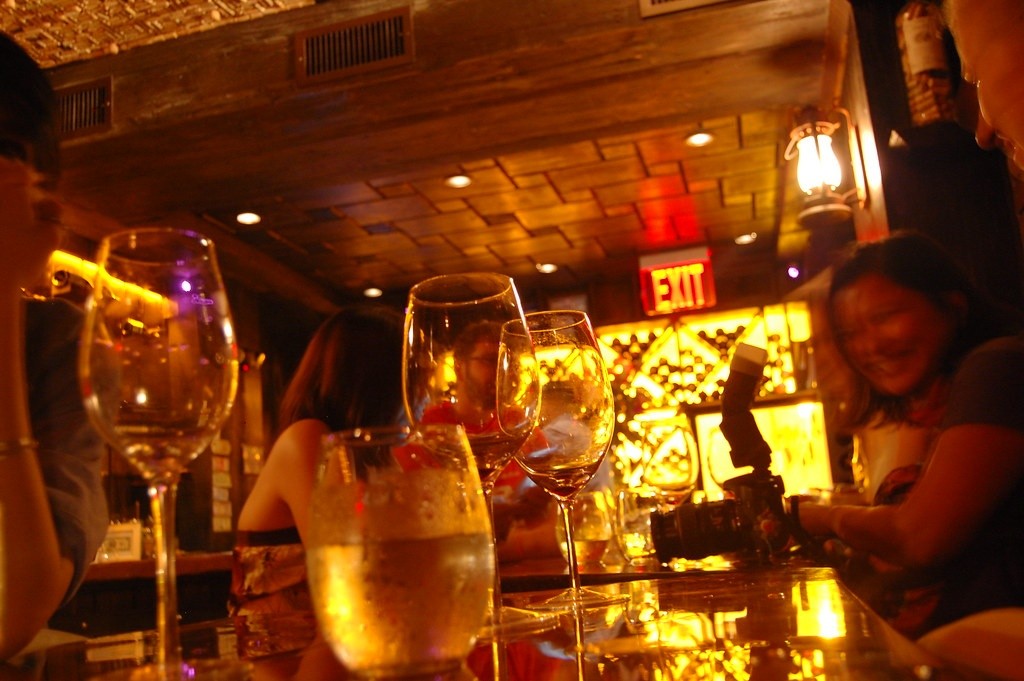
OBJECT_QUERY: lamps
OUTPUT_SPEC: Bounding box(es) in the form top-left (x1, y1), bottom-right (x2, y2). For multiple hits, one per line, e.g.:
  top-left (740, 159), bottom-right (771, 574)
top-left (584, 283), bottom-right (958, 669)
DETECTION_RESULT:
top-left (782, 103), bottom-right (869, 232)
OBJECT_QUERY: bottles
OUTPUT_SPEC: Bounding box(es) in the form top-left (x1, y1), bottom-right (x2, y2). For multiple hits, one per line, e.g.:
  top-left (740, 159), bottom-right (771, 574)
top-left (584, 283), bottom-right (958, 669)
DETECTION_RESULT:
top-left (613, 326), bottom-right (787, 401)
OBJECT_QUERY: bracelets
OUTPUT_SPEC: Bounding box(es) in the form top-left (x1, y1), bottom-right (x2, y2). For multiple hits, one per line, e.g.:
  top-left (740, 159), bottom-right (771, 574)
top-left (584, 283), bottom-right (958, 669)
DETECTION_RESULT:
top-left (0, 438), bottom-right (40, 453)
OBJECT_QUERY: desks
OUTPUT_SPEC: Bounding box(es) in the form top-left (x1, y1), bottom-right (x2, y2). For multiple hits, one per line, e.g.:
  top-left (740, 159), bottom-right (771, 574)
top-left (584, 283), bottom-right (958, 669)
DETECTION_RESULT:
top-left (94, 567), bottom-right (930, 681)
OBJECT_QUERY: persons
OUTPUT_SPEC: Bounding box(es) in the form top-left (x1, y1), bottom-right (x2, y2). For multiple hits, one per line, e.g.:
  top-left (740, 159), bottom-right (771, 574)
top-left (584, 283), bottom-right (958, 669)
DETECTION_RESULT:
top-left (783, 228), bottom-right (1024, 642)
top-left (886, 1), bottom-right (1024, 680)
top-left (227, 311), bottom-right (599, 659)
top-left (0, 32), bottom-right (110, 681)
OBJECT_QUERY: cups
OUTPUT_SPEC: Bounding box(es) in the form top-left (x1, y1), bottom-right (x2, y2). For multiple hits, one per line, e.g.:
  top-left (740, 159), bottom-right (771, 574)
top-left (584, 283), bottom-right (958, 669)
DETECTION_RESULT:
top-left (303, 421), bottom-right (493, 681)
top-left (557, 491), bottom-right (614, 565)
top-left (616, 492), bottom-right (658, 568)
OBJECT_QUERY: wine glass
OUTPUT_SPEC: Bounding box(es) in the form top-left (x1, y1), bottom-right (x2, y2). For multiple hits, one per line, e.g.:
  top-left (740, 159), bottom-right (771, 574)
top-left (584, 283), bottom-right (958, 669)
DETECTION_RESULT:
top-left (402, 271), bottom-right (560, 639)
top-left (76, 228), bottom-right (257, 681)
top-left (495, 309), bottom-right (633, 610)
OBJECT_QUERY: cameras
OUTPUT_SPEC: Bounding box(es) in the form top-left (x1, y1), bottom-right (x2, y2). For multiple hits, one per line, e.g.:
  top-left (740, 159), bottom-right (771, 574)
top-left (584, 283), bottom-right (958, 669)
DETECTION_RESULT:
top-left (649, 342), bottom-right (792, 564)
